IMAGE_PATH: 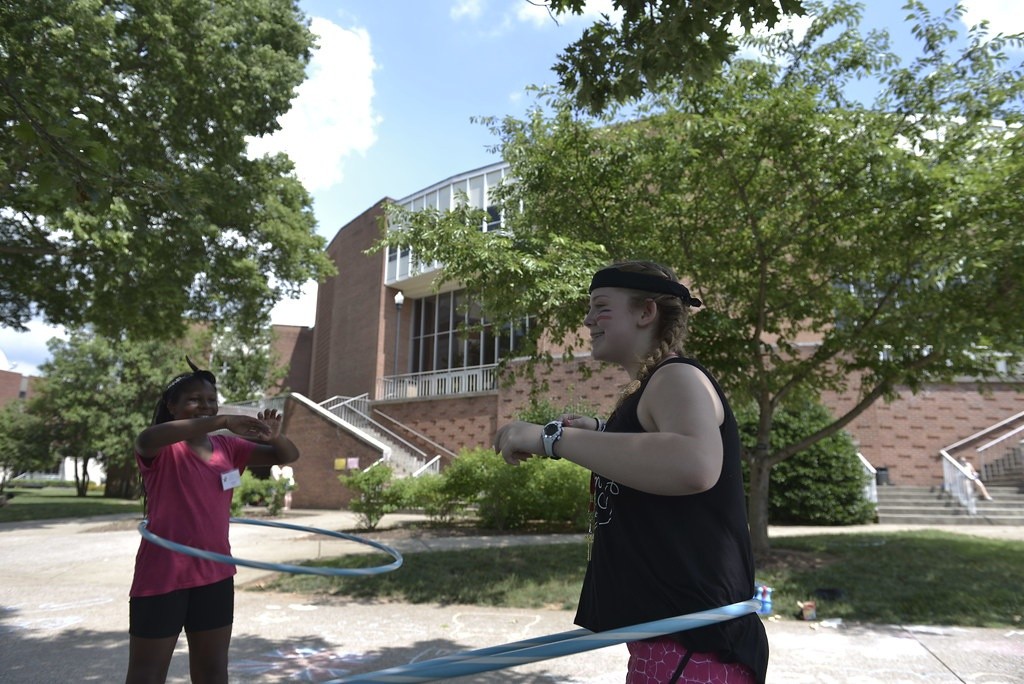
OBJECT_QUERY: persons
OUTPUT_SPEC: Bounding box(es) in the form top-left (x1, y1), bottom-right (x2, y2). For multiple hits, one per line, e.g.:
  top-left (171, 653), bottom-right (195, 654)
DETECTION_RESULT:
top-left (494, 261), bottom-right (770, 684)
top-left (126, 368), bottom-right (300, 684)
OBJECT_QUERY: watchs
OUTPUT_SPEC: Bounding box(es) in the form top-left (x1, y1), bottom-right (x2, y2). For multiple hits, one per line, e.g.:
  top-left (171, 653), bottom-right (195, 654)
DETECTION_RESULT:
top-left (542, 421), bottom-right (566, 460)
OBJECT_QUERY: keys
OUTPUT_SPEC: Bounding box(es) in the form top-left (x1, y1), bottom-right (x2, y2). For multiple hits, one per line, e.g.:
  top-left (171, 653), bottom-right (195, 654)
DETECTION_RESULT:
top-left (585, 532), bottom-right (594, 561)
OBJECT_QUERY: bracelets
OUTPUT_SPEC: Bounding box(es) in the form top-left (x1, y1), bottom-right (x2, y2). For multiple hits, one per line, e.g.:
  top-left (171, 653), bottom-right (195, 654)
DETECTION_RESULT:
top-left (595, 416), bottom-right (606, 432)
